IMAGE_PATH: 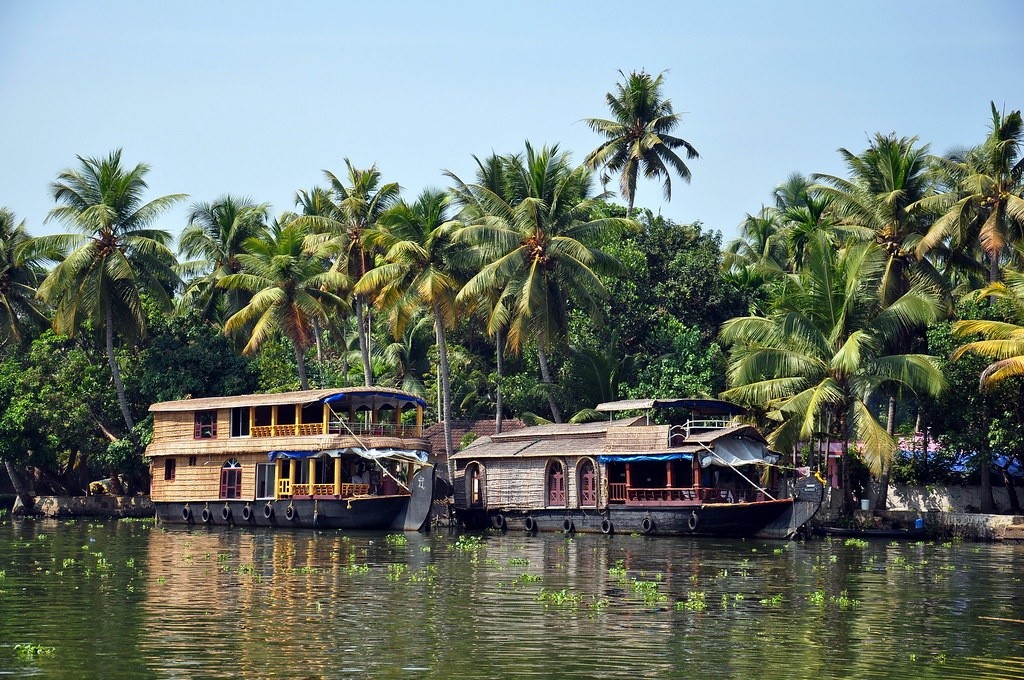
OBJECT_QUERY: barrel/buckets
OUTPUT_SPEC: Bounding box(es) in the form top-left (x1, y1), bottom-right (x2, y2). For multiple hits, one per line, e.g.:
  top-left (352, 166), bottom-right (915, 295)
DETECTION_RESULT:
top-left (915, 518), bottom-right (923, 529)
top-left (861, 499), bottom-right (870, 510)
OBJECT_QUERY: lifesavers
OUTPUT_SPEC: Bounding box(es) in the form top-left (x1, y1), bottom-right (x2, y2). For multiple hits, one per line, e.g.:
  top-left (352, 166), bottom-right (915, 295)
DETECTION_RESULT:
top-left (642, 518), bottom-right (652, 533)
top-left (473, 513), bottom-right (481, 525)
top-left (495, 514), bottom-right (504, 528)
top-left (242, 506), bottom-right (253, 520)
top-left (263, 504), bottom-right (273, 519)
top-left (285, 506), bottom-right (295, 520)
top-left (601, 520), bottom-right (611, 534)
top-left (222, 507), bottom-right (231, 520)
top-left (525, 517), bottom-right (534, 530)
top-left (688, 514), bottom-right (698, 530)
top-left (182, 507), bottom-right (191, 521)
top-left (202, 509), bottom-right (210, 522)
top-left (563, 518), bottom-right (572, 531)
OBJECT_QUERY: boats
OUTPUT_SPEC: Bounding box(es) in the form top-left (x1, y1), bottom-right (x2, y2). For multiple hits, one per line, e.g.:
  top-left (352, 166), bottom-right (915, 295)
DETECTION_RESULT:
top-left (143, 388), bottom-right (456, 533)
top-left (815, 515), bottom-right (928, 540)
top-left (448, 397), bottom-right (799, 540)
